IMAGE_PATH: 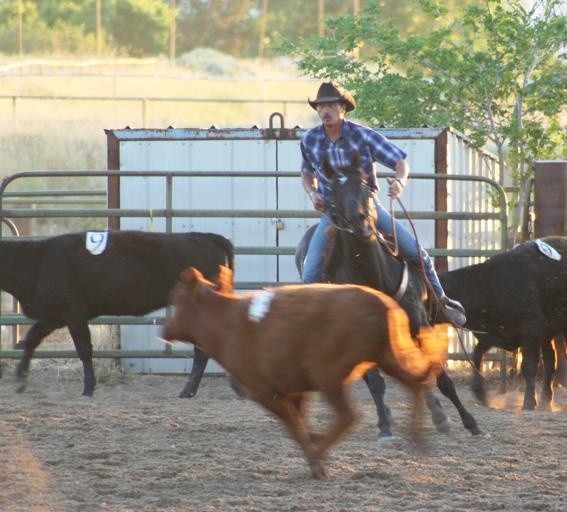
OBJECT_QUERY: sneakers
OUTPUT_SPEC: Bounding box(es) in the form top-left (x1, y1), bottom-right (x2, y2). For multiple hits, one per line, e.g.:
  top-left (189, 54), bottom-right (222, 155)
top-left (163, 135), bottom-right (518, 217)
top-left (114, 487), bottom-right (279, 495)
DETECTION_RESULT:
top-left (443, 303), bottom-right (466, 327)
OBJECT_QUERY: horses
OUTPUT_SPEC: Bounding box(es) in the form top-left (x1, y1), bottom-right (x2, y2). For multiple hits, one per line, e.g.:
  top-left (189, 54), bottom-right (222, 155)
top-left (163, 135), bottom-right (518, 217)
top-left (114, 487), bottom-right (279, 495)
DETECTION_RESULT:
top-left (294, 148), bottom-right (483, 444)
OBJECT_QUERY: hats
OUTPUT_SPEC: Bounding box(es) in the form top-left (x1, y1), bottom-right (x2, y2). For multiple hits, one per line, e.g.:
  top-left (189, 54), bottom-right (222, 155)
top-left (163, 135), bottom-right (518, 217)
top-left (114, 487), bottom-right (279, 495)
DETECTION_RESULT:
top-left (308, 81), bottom-right (356, 112)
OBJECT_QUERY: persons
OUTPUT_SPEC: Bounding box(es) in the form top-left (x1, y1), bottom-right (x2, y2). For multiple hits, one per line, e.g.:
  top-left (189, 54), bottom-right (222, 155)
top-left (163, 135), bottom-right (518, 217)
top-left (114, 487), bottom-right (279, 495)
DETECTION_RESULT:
top-left (301, 78), bottom-right (468, 328)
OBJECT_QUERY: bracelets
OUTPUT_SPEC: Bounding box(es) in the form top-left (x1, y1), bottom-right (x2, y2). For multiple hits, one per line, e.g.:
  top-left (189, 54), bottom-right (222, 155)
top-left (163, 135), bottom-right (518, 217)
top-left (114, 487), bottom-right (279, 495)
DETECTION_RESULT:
top-left (390, 175), bottom-right (406, 189)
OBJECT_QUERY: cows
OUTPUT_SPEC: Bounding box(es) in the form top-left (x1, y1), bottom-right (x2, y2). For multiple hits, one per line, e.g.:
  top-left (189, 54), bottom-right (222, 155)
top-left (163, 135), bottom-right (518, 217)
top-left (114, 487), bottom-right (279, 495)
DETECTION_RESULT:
top-left (156, 262), bottom-right (443, 483)
top-left (438, 233), bottom-right (567, 414)
top-left (0, 226), bottom-right (250, 400)
